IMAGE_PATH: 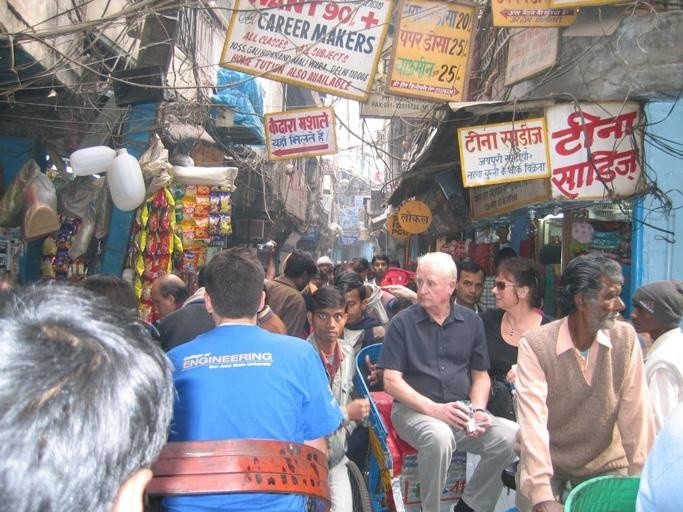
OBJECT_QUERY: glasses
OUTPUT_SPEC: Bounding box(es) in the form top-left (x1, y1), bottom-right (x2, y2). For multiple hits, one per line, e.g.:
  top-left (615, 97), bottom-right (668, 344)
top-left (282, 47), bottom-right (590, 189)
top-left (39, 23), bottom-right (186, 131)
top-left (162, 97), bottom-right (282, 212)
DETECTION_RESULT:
top-left (497, 281), bottom-right (516, 291)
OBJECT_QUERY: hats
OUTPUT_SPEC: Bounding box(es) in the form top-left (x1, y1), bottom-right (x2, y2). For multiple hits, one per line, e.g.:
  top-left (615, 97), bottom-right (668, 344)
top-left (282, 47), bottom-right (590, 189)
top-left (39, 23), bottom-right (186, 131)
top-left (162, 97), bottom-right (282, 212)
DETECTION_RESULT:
top-left (316, 256), bottom-right (333, 267)
top-left (632, 282), bottom-right (683, 326)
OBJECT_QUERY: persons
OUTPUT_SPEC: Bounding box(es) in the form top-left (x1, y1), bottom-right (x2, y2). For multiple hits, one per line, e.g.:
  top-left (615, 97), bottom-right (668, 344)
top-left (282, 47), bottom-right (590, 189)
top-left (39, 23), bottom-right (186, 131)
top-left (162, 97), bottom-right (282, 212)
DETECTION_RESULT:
top-left (2, 247), bottom-right (682, 512)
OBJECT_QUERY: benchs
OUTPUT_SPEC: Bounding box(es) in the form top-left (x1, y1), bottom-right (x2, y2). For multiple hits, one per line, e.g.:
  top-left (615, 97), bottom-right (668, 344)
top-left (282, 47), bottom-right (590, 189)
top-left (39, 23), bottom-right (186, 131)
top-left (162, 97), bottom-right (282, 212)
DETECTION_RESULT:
top-left (369, 389), bottom-right (485, 511)
